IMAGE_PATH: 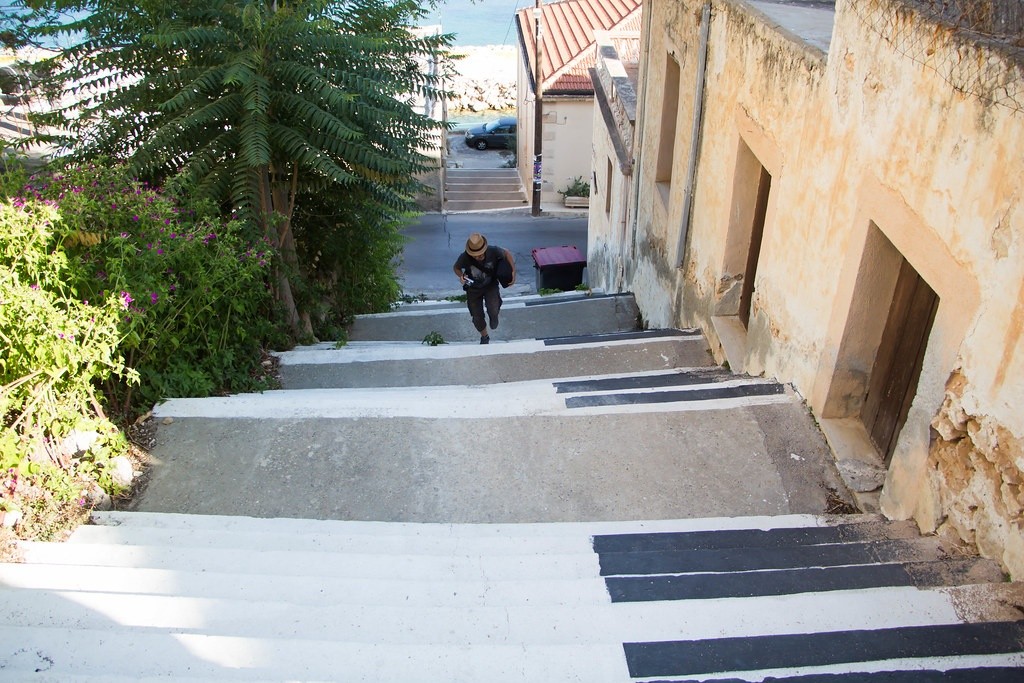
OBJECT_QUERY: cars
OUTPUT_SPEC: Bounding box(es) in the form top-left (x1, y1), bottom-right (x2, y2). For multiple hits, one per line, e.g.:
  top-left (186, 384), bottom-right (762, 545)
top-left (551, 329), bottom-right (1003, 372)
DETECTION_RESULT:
top-left (464, 116), bottom-right (517, 151)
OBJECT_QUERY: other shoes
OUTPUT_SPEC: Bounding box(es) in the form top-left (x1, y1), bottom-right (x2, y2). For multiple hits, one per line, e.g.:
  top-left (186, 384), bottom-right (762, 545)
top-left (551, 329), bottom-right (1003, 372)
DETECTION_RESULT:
top-left (480, 334), bottom-right (490, 344)
top-left (490, 315), bottom-right (498, 329)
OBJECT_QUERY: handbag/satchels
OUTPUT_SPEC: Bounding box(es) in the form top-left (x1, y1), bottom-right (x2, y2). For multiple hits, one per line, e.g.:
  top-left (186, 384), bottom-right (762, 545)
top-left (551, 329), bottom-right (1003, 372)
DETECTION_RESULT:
top-left (496, 259), bottom-right (512, 288)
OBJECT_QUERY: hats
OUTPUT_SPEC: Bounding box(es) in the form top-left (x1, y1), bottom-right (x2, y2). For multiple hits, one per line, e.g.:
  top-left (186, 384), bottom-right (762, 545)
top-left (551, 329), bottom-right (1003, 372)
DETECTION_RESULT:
top-left (465, 233), bottom-right (488, 256)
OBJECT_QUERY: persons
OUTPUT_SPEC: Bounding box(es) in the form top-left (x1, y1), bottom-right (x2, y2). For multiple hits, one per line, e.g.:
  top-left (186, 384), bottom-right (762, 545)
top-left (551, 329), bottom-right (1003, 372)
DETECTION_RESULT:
top-left (452, 231), bottom-right (516, 344)
